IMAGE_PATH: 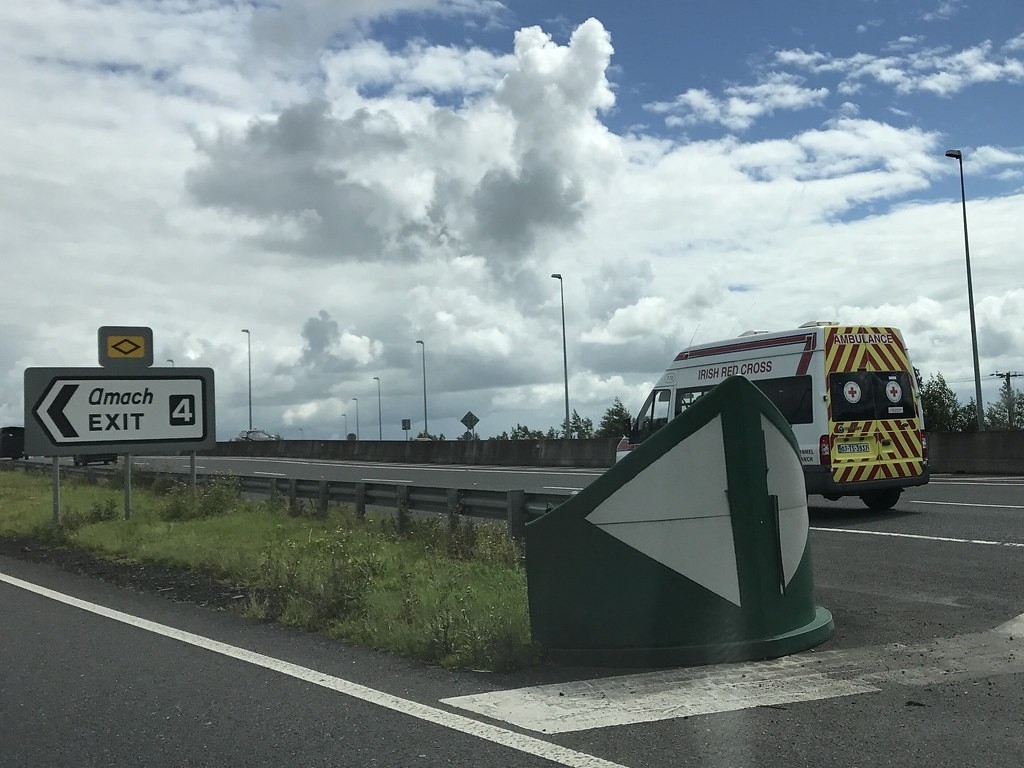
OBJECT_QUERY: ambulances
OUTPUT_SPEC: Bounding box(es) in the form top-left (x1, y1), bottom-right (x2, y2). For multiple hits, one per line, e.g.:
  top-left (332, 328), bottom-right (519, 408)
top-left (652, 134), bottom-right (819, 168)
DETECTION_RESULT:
top-left (614, 319), bottom-right (931, 513)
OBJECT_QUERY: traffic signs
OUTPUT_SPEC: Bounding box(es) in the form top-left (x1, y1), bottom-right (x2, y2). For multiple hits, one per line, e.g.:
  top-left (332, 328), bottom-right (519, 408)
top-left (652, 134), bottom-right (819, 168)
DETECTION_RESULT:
top-left (30, 371), bottom-right (211, 447)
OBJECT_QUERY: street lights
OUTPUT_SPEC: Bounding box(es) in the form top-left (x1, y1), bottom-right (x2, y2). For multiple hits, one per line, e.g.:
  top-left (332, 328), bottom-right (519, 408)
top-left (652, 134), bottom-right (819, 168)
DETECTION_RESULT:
top-left (351, 398), bottom-right (359, 439)
top-left (241, 328), bottom-right (253, 429)
top-left (298, 428), bottom-right (305, 440)
top-left (414, 339), bottom-right (429, 436)
top-left (342, 412), bottom-right (350, 439)
top-left (551, 272), bottom-right (571, 435)
top-left (373, 377), bottom-right (382, 441)
top-left (945, 149), bottom-right (985, 434)
top-left (166, 359), bottom-right (175, 368)
